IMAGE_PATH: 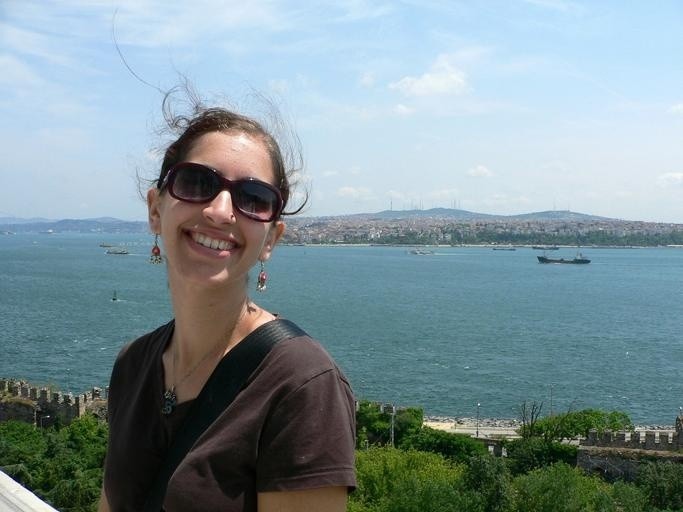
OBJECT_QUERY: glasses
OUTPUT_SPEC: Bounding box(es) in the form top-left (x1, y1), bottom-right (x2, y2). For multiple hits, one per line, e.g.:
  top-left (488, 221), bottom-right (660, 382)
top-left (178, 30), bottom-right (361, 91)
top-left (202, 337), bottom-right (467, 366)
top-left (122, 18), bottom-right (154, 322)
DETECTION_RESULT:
top-left (162, 162), bottom-right (283, 223)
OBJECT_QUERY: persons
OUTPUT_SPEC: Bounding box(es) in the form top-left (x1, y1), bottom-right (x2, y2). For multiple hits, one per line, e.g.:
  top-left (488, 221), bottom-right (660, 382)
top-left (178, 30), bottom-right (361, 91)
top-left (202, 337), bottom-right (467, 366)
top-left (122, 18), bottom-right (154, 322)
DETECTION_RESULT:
top-left (97, 75), bottom-right (357, 512)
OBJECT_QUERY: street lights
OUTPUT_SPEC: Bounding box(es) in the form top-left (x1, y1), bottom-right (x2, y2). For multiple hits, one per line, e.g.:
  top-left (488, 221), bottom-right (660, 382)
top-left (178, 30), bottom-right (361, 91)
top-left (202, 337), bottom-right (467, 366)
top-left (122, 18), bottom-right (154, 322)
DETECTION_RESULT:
top-left (476, 402), bottom-right (481, 437)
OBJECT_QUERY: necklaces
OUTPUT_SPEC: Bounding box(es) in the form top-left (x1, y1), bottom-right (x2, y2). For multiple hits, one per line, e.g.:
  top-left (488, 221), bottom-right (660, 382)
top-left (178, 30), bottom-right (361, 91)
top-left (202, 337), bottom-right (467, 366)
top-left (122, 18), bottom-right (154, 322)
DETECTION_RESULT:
top-left (161, 306), bottom-right (249, 416)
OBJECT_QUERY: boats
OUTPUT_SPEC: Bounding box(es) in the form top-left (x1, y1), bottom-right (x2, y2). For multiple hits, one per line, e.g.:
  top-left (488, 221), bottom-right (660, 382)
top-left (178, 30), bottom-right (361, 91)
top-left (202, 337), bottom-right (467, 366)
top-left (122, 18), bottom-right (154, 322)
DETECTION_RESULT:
top-left (405, 246), bottom-right (434, 257)
top-left (537, 252), bottom-right (591, 263)
top-left (99, 242), bottom-right (130, 254)
top-left (493, 248), bottom-right (517, 250)
top-left (532, 243), bottom-right (559, 249)
